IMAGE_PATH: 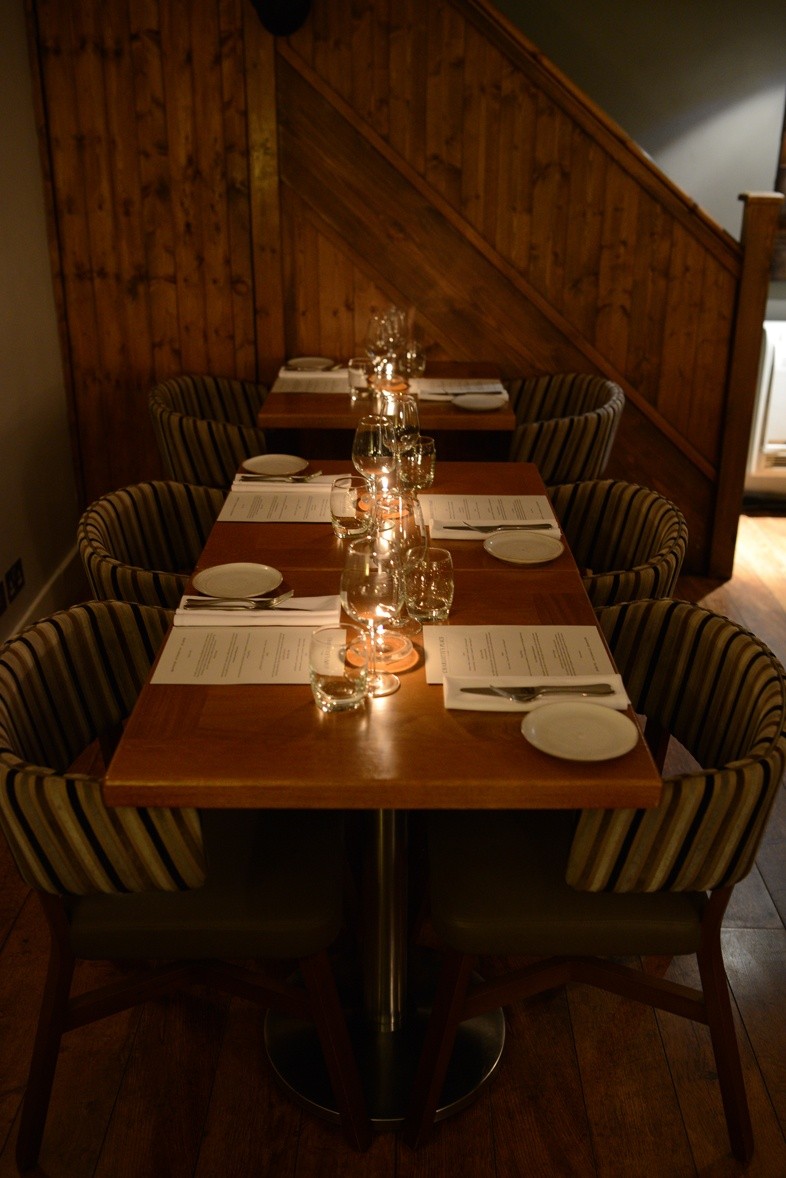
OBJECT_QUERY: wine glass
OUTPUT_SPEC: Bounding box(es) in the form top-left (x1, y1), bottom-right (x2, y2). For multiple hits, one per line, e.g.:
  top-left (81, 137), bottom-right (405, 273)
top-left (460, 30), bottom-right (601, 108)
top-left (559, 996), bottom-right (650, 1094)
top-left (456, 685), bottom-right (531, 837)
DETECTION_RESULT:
top-left (331, 305), bottom-right (457, 704)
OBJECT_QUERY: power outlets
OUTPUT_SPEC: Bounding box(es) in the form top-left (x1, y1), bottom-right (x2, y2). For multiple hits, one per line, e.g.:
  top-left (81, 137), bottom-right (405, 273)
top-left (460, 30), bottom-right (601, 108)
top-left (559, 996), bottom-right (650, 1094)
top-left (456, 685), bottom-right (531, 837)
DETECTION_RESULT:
top-left (4, 556), bottom-right (24, 604)
top-left (0, 578), bottom-right (9, 617)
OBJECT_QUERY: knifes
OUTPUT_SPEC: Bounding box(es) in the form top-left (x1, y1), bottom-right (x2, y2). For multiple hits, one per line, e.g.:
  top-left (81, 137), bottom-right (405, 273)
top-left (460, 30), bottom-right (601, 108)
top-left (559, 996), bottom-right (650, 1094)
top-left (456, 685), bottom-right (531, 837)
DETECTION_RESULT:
top-left (240, 478), bottom-right (332, 484)
top-left (185, 604), bottom-right (315, 611)
top-left (429, 391), bottom-right (502, 396)
top-left (458, 684), bottom-right (610, 697)
top-left (444, 524), bottom-right (553, 530)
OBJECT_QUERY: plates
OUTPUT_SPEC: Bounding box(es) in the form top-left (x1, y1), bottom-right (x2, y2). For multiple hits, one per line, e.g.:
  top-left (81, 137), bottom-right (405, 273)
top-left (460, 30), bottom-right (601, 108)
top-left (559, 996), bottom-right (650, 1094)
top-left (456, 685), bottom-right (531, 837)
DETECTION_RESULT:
top-left (451, 394), bottom-right (507, 411)
top-left (242, 454), bottom-right (309, 474)
top-left (193, 563), bottom-right (282, 599)
top-left (520, 701), bottom-right (639, 764)
top-left (287, 356), bottom-right (333, 369)
top-left (482, 530), bottom-right (565, 565)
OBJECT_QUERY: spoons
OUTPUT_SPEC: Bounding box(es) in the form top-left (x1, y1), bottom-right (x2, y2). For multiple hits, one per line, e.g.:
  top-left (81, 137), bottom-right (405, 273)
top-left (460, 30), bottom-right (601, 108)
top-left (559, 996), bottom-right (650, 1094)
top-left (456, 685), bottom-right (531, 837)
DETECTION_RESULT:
top-left (284, 362), bottom-right (343, 371)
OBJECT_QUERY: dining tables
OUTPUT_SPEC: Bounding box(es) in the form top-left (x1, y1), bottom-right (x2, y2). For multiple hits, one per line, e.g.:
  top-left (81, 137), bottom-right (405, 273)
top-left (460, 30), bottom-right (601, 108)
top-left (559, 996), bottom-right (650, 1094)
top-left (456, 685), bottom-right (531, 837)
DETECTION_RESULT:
top-left (97, 461), bottom-right (663, 1137)
top-left (254, 354), bottom-right (519, 463)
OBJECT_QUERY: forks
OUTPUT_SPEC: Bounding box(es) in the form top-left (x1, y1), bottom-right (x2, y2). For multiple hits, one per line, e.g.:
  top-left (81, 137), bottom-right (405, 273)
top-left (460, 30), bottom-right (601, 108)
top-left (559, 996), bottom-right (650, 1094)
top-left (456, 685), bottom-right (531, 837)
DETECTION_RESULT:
top-left (241, 470), bottom-right (323, 481)
top-left (463, 521), bottom-right (551, 532)
top-left (186, 589), bottom-right (295, 607)
top-left (490, 685), bottom-right (615, 701)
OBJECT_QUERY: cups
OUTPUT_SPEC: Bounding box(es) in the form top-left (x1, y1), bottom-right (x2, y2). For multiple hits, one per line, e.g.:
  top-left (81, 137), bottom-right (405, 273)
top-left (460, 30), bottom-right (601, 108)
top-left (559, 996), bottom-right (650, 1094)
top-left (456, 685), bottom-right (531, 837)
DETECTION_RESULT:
top-left (308, 623), bottom-right (368, 712)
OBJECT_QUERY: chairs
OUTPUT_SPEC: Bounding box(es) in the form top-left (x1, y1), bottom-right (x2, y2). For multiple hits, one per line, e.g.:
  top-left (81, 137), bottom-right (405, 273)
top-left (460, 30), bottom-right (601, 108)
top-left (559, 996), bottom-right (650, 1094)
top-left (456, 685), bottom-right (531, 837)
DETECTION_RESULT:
top-left (75, 478), bottom-right (229, 615)
top-left (145, 372), bottom-right (274, 497)
top-left (396, 596), bottom-right (786, 1170)
top-left (527, 479), bottom-right (690, 608)
top-left (0, 599), bottom-right (388, 1177)
top-left (503, 370), bottom-right (629, 492)
